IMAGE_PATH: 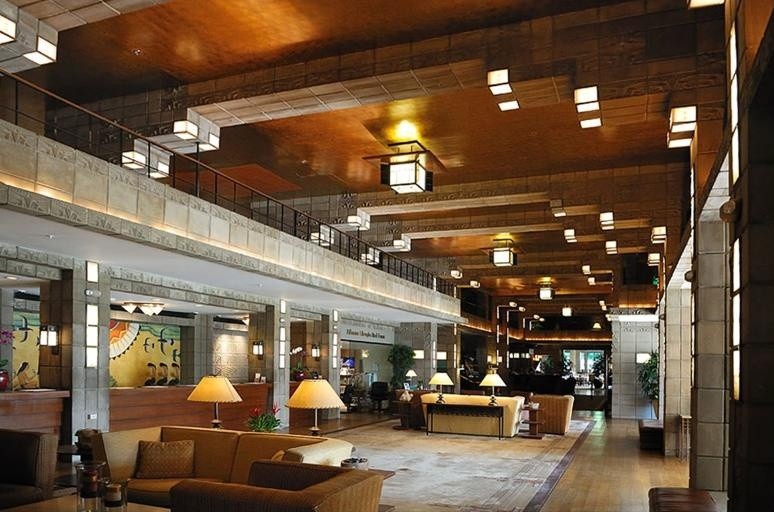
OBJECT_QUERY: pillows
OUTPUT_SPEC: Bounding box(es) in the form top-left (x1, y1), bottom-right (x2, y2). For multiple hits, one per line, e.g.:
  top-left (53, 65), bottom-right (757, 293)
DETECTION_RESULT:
top-left (272, 449), bottom-right (285, 460)
top-left (131, 440), bottom-right (194, 478)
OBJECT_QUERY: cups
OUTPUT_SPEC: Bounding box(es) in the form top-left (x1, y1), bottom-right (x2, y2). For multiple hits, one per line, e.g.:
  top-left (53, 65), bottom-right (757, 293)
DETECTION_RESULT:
top-left (75, 461), bottom-right (106, 512)
top-left (96, 477), bottom-right (128, 512)
top-left (255, 373), bottom-right (260, 381)
top-left (261, 376), bottom-right (266, 383)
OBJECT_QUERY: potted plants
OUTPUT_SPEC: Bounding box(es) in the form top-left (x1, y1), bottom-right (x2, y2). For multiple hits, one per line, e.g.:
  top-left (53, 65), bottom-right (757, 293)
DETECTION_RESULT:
top-left (631, 350), bottom-right (659, 418)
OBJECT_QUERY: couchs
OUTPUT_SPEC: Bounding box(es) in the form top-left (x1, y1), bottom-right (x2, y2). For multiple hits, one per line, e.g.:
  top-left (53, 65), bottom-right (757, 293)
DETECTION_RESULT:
top-left (0, 429), bottom-right (58, 512)
top-left (77, 424), bottom-right (353, 506)
top-left (170, 459), bottom-right (395, 512)
top-left (511, 373), bottom-right (577, 394)
top-left (420, 393), bottom-right (525, 437)
top-left (396, 387), bottom-right (443, 425)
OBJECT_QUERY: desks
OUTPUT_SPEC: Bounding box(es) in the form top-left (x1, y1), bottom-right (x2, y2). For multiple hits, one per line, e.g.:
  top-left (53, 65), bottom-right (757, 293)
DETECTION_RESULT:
top-left (426, 401), bottom-right (505, 440)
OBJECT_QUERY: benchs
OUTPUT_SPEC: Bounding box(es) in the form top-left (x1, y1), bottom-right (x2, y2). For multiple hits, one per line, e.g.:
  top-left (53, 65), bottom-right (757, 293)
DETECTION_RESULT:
top-left (638, 419), bottom-right (664, 453)
top-left (649, 487), bottom-right (719, 512)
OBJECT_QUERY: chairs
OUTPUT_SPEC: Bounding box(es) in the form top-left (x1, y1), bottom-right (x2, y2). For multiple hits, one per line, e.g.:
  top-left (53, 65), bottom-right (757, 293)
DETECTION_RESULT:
top-left (510, 391), bottom-right (533, 404)
top-left (371, 381), bottom-right (390, 414)
top-left (530, 394), bottom-right (575, 435)
top-left (341, 383), bottom-right (353, 409)
top-left (463, 390), bottom-right (485, 395)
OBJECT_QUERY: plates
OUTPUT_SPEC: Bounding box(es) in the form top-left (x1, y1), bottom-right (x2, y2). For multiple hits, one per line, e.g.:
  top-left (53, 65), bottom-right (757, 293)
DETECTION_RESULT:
top-left (245, 383), bottom-right (263, 384)
top-left (143, 386), bottom-right (168, 389)
top-left (176, 385), bottom-right (196, 387)
top-left (20, 389), bottom-right (56, 392)
top-left (110, 387), bottom-right (136, 389)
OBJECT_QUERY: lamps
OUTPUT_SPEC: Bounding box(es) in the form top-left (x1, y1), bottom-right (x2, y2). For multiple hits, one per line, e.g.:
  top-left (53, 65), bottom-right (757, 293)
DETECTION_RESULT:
top-left (286, 374), bottom-right (344, 435)
top-left (429, 373), bottom-right (453, 404)
top-left (311, 343), bottom-right (321, 362)
top-left (188, 371), bottom-right (237, 428)
top-left (406, 370), bottom-right (417, 387)
top-left (479, 372), bottom-right (507, 406)
top-left (252, 341), bottom-right (264, 360)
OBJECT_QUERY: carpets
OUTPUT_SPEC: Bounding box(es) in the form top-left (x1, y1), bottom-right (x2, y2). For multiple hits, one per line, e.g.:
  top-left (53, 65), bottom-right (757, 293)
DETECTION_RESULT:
top-left (317, 414), bottom-right (596, 512)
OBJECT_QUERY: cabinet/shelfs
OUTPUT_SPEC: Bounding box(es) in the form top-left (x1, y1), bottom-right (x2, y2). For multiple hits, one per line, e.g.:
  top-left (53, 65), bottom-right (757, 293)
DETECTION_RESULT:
top-left (520, 406), bottom-right (547, 440)
top-left (368, 468), bottom-right (395, 512)
top-left (391, 401), bottom-right (415, 430)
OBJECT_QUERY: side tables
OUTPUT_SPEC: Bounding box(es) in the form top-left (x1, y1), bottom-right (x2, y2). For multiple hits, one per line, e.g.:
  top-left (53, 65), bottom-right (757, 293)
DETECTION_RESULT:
top-left (55, 445), bottom-right (79, 487)
